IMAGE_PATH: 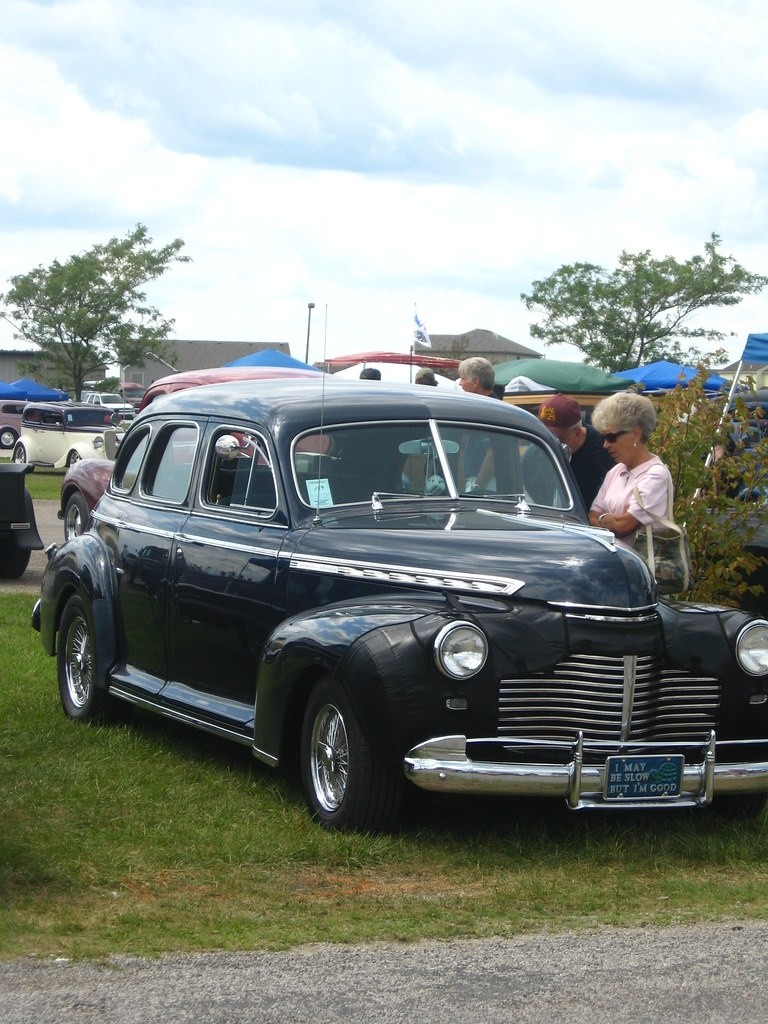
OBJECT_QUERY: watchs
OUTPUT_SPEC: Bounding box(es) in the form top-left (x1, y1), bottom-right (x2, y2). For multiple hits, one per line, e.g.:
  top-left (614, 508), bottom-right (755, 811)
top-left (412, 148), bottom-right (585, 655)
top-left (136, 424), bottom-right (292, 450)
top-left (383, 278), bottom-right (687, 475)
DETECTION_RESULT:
top-left (473, 484), bottom-right (481, 489)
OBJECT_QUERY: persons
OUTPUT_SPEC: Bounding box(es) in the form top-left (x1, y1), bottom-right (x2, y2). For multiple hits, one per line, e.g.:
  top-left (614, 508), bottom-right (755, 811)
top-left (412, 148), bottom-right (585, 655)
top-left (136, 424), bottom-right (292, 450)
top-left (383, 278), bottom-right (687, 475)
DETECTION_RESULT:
top-left (338, 358), bottom-right (520, 494)
top-left (522, 393), bottom-right (616, 512)
top-left (587, 393), bottom-right (674, 601)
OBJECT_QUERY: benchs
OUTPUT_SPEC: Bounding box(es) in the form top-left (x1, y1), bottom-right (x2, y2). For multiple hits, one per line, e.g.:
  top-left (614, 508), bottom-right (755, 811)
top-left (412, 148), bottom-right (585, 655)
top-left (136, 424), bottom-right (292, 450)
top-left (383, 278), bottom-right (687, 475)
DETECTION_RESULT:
top-left (175, 464), bottom-right (394, 517)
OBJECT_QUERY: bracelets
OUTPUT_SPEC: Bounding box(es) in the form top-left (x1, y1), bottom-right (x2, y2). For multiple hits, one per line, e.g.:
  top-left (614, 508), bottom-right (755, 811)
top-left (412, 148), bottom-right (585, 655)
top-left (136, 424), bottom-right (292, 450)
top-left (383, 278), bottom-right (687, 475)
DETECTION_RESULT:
top-left (598, 513), bottom-right (610, 520)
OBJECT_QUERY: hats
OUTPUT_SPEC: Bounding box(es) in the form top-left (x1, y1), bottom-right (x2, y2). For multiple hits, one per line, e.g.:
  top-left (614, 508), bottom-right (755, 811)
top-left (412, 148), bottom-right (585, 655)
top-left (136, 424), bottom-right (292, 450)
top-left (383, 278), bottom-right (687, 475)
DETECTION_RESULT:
top-left (359, 367), bottom-right (381, 382)
top-left (416, 367), bottom-right (439, 386)
top-left (534, 390), bottom-right (583, 429)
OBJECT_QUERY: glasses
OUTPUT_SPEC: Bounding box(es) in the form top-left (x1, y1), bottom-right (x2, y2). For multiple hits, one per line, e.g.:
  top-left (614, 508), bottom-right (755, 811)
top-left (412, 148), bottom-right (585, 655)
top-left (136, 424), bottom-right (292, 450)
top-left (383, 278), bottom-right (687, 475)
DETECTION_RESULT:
top-left (599, 430), bottom-right (627, 444)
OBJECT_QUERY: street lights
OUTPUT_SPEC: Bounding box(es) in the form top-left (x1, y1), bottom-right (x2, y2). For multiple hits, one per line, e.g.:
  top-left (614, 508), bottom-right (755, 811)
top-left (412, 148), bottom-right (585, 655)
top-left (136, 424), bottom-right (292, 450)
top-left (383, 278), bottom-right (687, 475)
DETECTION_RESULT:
top-left (305, 302), bottom-right (316, 364)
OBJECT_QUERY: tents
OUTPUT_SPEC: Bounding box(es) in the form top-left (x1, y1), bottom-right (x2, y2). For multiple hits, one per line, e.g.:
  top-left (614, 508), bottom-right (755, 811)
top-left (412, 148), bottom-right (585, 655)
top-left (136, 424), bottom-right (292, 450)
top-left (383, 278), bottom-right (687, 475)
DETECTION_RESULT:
top-left (325, 350), bottom-right (464, 374)
top-left (611, 333), bottom-right (768, 531)
top-left (0, 377), bottom-right (68, 402)
top-left (492, 359), bottom-right (637, 394)
top-left (221, 348), bottom-right (321, 371)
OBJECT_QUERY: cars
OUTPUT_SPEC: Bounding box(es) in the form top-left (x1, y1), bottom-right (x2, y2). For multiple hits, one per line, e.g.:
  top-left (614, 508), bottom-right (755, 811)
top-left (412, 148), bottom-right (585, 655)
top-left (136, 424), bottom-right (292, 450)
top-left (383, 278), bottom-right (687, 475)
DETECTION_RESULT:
top-left (81, 379), bottom-right (105, 392)
top-left (80, 392), bottom-right (136, 422)
top-left (31, 369), bottom-right (768, 835)
top-left (1, 397), bottom-right (42, 449)
top-left (57, 365), bottom-right (431, 545)
top-left (117, 380), bottom-right (147, 412)
top-left (10, 400), bottom-right (130, 473)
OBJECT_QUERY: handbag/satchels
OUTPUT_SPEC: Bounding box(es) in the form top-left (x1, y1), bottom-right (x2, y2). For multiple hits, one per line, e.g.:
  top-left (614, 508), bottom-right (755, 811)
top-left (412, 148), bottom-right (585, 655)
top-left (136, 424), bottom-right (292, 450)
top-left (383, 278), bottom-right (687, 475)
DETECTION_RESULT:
top-left (629, 465), bottom-right (693, 598)
